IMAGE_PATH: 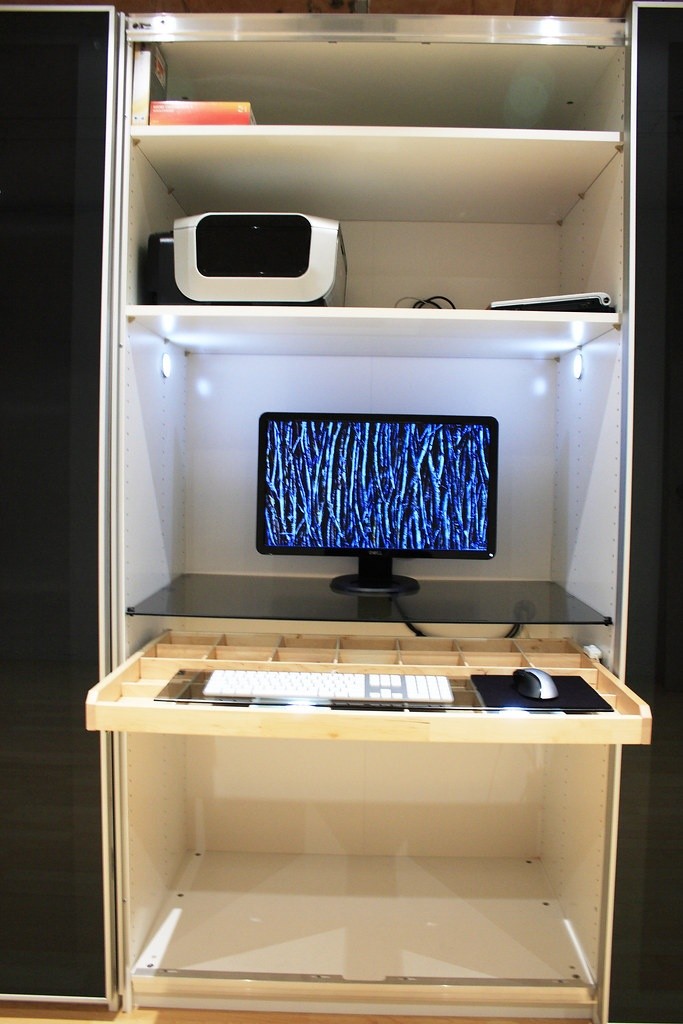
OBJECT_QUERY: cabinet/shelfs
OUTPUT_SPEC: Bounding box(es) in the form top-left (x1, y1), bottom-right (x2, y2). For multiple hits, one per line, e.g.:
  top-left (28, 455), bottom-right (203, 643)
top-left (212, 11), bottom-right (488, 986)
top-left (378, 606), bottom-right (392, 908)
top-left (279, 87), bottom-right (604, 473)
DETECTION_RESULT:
top-left (83, 11), bottom-right (656, 1024)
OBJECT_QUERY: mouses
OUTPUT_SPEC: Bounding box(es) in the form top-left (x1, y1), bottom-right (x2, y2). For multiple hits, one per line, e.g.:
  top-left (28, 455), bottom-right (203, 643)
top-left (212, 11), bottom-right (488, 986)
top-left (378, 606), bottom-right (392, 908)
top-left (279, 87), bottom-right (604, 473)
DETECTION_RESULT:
top-left (513, 668), bottom-right (559, 700)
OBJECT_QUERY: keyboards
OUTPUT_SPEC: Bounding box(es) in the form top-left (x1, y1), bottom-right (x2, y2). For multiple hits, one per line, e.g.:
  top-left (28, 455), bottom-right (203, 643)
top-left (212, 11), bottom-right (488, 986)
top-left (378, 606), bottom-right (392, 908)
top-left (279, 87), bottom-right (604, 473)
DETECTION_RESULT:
top-left (203, 669), bottom-right (454, 704)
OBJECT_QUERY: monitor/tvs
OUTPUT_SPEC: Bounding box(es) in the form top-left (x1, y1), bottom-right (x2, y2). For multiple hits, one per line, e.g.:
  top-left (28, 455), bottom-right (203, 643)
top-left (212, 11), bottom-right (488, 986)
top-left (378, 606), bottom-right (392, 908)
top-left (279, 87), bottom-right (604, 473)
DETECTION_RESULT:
top-left (255, 413), bottom-right (498, 595)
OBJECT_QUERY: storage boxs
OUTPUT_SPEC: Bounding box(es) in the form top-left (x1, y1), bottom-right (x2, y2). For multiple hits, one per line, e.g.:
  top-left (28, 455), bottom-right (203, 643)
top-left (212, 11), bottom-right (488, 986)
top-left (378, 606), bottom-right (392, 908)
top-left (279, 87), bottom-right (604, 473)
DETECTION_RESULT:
top-left (149, 100), bottom-right (257, 126)
top-left (132, 41), bottom-right (168, 127)
